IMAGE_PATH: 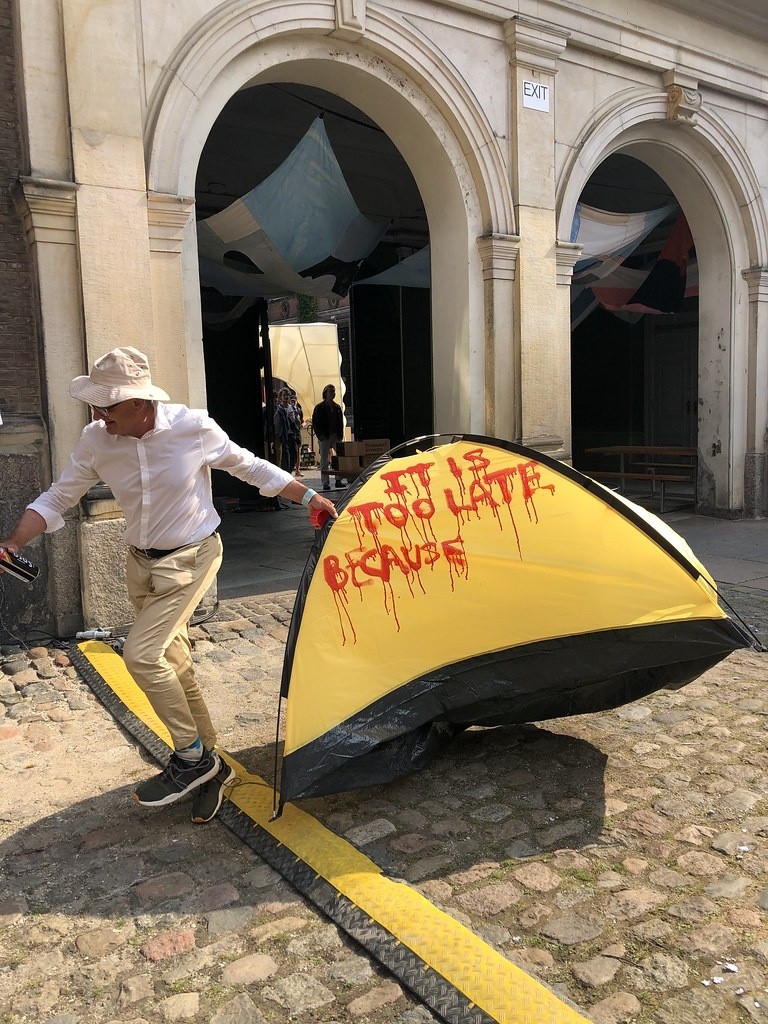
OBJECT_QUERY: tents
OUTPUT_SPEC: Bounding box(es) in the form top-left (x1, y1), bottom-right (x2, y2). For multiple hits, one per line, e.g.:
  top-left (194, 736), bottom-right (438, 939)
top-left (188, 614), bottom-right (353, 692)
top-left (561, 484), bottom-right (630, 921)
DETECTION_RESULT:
top-left (273, 432), bottom-right (768, 821)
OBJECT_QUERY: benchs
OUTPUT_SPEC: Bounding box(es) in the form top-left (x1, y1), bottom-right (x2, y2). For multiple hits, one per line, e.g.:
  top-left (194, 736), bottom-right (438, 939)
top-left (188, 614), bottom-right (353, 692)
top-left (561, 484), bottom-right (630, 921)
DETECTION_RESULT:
top-left (580, 470), bottom-right (693, 514)
top-left (630, 463), bottom-right (696, 496)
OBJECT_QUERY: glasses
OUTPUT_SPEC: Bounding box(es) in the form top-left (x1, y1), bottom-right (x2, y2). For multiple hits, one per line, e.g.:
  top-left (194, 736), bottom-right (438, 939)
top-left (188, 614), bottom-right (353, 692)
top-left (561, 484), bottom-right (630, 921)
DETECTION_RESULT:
top-left (87, 402), bottom-right (121, 417)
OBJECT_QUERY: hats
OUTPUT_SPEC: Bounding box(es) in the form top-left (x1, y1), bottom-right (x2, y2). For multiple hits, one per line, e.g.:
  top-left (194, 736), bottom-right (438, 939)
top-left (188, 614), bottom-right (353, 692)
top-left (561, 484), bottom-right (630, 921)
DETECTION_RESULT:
top-left (322, 384), bottom-right (335, 399)
top-left (289, 395), bottom-right (297, 401)
top-left (69, 347), bottom-right (170, 407)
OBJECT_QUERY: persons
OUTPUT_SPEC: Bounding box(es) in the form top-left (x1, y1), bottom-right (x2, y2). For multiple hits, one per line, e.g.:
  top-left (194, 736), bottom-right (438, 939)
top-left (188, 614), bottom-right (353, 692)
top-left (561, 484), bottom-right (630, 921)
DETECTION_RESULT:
top-left (0, 347), bottom-right (338, 823)
top-left (261, 386), bottom-right (305, 477)
top-left (312, 384), bottom-right (346, 490)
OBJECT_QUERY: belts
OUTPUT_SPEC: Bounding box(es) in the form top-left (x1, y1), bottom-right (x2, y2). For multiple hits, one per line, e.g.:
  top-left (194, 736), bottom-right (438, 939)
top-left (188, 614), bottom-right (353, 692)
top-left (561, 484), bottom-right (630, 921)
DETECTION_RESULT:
top-left (134, 532), bottom-right (215, 559)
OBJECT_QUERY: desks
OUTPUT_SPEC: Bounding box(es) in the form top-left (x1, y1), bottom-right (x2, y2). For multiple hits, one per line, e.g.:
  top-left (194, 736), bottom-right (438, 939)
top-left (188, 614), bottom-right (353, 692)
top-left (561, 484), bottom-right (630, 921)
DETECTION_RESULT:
top-left (584, 445), bottom-right (698, 505)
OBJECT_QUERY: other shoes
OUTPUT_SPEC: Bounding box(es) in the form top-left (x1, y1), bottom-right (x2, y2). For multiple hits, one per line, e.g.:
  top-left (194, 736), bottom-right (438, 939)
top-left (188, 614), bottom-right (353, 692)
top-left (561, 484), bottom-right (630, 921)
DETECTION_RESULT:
top-left (323, 485), bottom-right (330, 490)
top-left (294, 470), bottom-right (304, 477)
top-left (335, 482), bottom-right (346, 488)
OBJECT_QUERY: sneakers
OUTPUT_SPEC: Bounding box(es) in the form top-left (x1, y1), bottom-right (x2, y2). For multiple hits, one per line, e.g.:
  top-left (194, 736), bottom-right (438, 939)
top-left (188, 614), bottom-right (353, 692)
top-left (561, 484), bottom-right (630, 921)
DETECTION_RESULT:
top-left (191, 755), bottom-right (237, 822)
top-left (134, 745), bottom-right (218, 806)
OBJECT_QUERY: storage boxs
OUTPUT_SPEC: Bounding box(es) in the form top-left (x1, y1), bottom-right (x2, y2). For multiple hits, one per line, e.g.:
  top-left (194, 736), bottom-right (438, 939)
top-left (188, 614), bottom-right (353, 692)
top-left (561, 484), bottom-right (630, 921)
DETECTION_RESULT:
top-left (331, 439), bottom-right (391, 470)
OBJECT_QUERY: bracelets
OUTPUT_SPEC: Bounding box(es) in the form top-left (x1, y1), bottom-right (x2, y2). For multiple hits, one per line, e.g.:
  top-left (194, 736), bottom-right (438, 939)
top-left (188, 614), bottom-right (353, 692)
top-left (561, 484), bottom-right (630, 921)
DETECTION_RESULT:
top-left (302, 489), bottom-right (317, 508)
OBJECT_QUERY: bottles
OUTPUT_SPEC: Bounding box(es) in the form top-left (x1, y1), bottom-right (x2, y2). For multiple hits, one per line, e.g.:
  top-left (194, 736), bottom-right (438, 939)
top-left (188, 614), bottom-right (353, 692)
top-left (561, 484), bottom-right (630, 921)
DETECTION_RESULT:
top-left (1, 545), bottom-right (40, 584)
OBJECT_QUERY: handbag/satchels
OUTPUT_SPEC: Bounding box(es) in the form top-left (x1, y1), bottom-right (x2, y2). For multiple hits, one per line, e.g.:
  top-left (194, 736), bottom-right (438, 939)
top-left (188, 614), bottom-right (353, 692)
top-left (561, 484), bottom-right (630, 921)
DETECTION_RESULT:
top-left (291, 422), bottom-right (299, 434)
